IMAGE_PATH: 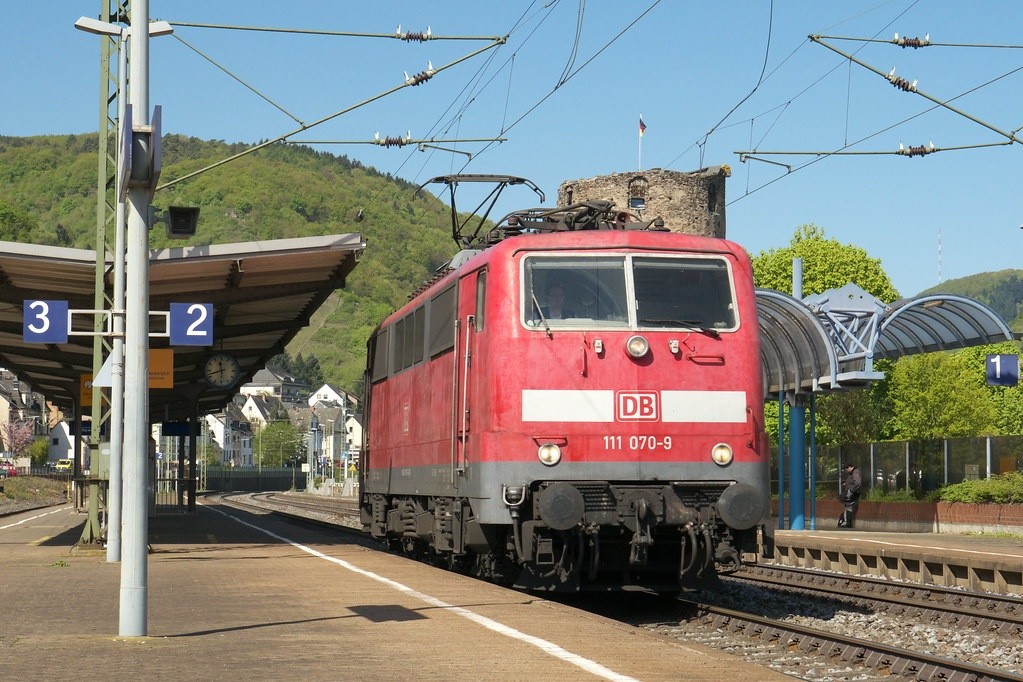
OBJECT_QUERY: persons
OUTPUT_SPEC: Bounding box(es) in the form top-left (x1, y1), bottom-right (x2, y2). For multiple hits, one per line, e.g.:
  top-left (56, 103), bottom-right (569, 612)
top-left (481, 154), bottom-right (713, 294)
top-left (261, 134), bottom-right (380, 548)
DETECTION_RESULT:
top-left (839, 463), bottom-right (862, 528)
top-left (531, 285), bottom-right (577, 320)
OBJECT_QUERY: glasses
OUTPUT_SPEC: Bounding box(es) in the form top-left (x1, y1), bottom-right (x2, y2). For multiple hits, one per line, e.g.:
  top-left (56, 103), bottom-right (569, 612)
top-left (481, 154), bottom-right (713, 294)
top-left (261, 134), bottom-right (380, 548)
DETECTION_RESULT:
top-left (549, 295), bottom-right (566, 299)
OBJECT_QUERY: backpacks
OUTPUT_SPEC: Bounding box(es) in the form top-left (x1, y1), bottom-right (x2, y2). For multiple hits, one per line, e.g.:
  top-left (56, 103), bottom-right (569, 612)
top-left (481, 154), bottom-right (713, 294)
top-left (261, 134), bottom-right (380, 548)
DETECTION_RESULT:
top-left (838, 510), bottom-right (852, 528)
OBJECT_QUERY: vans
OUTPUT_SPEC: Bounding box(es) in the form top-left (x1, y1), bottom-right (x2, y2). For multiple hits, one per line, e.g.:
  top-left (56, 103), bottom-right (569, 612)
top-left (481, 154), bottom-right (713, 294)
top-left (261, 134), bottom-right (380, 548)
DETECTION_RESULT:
top-left (55, 458), bottom-right (74, 473)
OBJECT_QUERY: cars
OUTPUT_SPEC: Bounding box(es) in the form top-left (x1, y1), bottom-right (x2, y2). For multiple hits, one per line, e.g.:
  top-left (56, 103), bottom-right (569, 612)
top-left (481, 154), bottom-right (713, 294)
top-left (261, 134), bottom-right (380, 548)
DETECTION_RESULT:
top-left (0, 462), bottom-right (17, 480)
top-left (823, 467), bottom-right (922, 489)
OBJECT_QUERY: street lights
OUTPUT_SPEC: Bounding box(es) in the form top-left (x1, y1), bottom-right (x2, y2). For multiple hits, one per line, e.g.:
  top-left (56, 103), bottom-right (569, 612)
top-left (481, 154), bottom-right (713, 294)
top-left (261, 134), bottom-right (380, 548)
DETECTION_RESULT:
top-left (319, 424), bottom-right (327, 492)
top-left (311, 427), bottom-right (318, 478)
top-left (327, 419), bottom-right (335, 496)
top-left (347, 413), bottom-right (355, 498)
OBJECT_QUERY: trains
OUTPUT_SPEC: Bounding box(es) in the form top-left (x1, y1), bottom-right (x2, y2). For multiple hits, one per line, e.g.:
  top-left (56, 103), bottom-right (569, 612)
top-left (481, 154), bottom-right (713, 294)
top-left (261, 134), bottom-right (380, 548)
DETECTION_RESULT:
top-left (360, 176), bottom-right (776, 618)
